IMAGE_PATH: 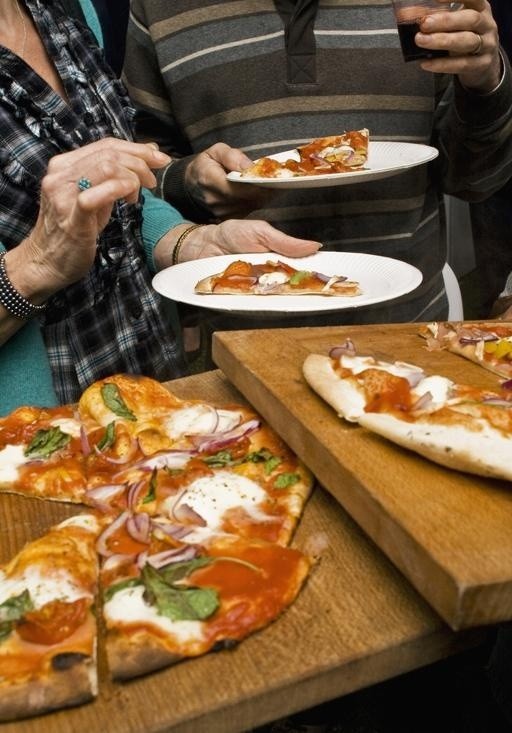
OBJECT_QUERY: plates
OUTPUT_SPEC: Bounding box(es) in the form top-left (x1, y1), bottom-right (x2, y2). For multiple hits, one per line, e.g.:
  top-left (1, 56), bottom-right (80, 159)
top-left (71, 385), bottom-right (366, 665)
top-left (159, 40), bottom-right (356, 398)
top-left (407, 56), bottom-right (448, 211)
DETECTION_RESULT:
top-left (226, 141), bottom-right (439, 189)
top-left (151, 251), bottom-right (424, 319)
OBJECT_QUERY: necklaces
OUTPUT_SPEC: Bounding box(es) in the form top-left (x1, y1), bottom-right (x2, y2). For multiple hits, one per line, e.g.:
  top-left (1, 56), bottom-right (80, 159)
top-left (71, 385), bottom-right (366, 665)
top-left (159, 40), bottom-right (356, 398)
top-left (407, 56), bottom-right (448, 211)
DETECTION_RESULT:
top-left (15, 0), bottom-right (26, 58)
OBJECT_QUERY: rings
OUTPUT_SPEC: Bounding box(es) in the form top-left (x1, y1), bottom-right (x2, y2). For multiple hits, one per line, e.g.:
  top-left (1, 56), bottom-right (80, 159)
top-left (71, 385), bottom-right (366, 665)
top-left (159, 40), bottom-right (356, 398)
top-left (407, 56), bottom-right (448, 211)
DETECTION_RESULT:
top-left (472, 33), bottom-right (482, 55)
top-left (77, 176), bottom-right (91, 191)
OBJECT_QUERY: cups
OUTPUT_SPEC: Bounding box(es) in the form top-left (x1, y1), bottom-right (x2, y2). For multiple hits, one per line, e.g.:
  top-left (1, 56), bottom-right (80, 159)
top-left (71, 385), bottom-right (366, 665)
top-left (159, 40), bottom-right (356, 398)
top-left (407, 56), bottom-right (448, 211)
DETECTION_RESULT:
top-left (392, 0), bottom-right (462, 66)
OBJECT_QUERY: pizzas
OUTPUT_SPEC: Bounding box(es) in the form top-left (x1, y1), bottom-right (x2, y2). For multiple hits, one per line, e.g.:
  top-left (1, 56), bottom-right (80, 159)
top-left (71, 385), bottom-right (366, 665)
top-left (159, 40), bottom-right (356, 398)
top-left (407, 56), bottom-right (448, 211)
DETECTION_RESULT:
top-left (1, 372), bottom-right (316, 722)
top-left (194, 258), bottom-right (362, 298)
top-left (238, 130), bottom-right (370, 179)
top-left (302, 320), bottom-right (512, 485)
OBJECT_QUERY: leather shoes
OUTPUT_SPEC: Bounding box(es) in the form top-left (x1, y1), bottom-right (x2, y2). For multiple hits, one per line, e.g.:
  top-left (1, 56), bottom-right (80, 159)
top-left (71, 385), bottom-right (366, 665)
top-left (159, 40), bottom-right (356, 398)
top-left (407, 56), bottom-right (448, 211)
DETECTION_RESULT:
top-left (184, 326), bottom-right (202, 364)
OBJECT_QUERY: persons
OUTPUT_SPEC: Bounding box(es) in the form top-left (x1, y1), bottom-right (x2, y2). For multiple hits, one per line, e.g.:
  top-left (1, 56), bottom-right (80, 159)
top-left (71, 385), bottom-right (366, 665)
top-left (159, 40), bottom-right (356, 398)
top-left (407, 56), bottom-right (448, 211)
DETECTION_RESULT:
top-left (0, 1), bottom-right (323, 417)
top-left (120, 0), bottom-right (511, 379)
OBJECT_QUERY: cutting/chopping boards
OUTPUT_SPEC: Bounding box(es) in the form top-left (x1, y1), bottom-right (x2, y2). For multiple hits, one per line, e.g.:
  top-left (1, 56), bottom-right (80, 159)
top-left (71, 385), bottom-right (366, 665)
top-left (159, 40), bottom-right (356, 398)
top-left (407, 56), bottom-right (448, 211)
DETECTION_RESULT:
top-left (0, 367), bottom-right (452, 733)
top-left (211, 320), bottom-right (512, 633)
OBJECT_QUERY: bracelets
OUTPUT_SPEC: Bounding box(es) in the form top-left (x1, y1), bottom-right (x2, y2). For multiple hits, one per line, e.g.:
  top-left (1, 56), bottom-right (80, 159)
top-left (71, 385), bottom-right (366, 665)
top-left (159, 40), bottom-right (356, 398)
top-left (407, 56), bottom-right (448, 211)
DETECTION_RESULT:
top-left (0, 250), bottom-right (48, 322)
top-left (172, 223), bottom-right (207, 265)
